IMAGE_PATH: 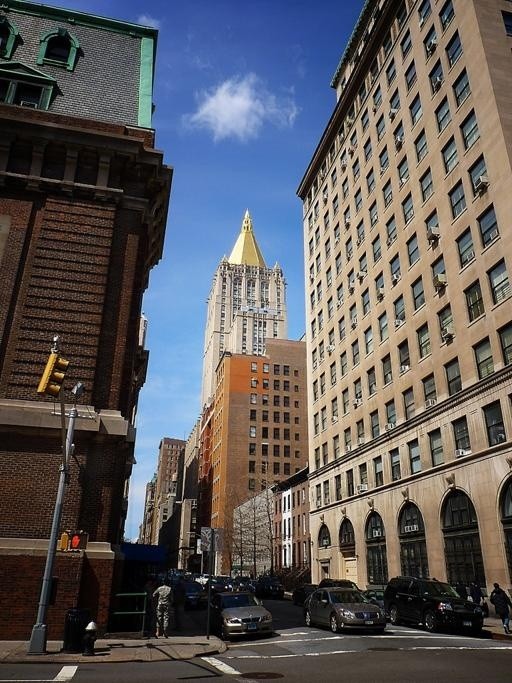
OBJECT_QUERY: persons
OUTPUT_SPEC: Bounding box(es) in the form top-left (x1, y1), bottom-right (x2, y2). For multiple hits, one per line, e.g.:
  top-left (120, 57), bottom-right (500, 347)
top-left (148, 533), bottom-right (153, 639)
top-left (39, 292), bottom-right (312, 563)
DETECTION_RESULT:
top-left (152, 577), bottom-right (175, 638)
top-left (456, 579), bottom-right (468, 600)
top-left (469, 581), bottom-right (484, 604)
top-left (490, 583), bottom-right (512, 634)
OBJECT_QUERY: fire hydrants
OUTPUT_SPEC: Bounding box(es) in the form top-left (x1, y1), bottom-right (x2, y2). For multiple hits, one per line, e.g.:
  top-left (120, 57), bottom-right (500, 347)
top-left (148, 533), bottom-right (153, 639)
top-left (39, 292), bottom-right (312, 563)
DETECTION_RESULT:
top-left (82, 620), bottom-right (98, 655)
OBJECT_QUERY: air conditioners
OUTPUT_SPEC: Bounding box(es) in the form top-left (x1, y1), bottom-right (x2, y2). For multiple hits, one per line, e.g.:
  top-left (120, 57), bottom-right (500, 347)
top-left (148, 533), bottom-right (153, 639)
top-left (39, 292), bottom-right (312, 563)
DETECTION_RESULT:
top-left (431, 272), bottom-right (447, 288)
top-left (466, 250), bottom-right (476, 262)
top-left (425, 39), bottom-right (436, 54)
top-left (349, 317), bottom-right (357, 328)
top-left (383, 422), bottom-right (394, 431)
top-left (394, 135), bottom-right (400, 149)
top-left (440, 326), bottom-right (454, 340)
top-left (398, 365), bottom-right (406, 373)
top-left (390, 274), bottom-right (399, 284)
top-left (334, 300), bottom-right (342, 307)
top-left (314, 500), bottom-right (321, 506)
top-left (345, 119), bottom-right (353, 127)
top-left (323, 498), bottom-right (331, 504)
top-left (391, 319), bottom-right (403, 328)
top-left (344, 445), bottom-right (351, 453)
top-left (317, 172), bottom-right (325, 181)
top-left (431, 75), bottom-right (441, 89)
top-left (346, 146), bottom-right (353, 154)
top-left (385, 236), bottom-right (392, 245)
top-left (355, 271), bottom-right (367, 279)
top-left (385, 194), bottom-right (394, 203)
top-left (375, 287), bottom-right (384, 300)
top-left (330, 415), bottom-right (338, 423)
top-left (354, 483), bottom-right (368, 492)
top-left (398, 178), bottom-right (406, 187)
top-left (473, 176), bottom-right (487, 192)
top-left (387, 108), bottom-right (396, 119)
top-left (408, 210), bottom-right (413, 217)
top-left (337, 133), bottom-right (345, 142)
top-left (370, 105), bottom-right (376, 112)
top-left (425, 226), bottom-right (439, 242)
top-left (338, 160), bottom-right (347, 166)
top-left (310, 272), bottom-right (315, 281)
top-left (355, 438), bottom-right (365, 446)
top-left (424, 398), bottom-right (436, 408)
top-left (346, 282), bottom-right (354, 290)
top-left (453, 448), bottom-right (466, 458)
top-left (351, 398), bottom-right (362, 406)
top-left (308, 194), bottom-right (364, 264)
top-left (371, 214), bottom-right (378, 224)
top-left (325, 345), bottom-right (334, 352)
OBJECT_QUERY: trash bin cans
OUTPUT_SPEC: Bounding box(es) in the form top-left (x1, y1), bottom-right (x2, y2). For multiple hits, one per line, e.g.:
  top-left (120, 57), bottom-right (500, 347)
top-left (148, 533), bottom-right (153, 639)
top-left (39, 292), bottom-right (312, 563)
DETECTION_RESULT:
top-left (63, 609), bottom-right (88, 654)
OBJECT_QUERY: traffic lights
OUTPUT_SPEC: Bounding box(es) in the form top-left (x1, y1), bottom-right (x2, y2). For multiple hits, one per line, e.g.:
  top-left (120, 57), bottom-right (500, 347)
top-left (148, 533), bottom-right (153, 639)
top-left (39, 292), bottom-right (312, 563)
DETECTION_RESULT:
top-left (70, 533), bottom-right (89, 550)
top-left (36, 352), bottom-right (71, 397)
